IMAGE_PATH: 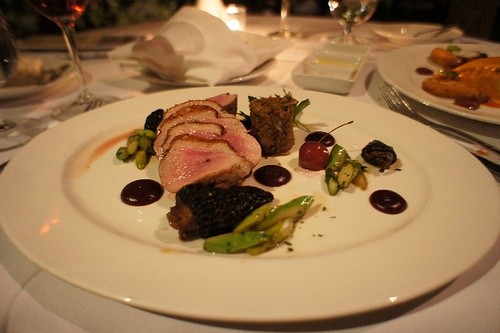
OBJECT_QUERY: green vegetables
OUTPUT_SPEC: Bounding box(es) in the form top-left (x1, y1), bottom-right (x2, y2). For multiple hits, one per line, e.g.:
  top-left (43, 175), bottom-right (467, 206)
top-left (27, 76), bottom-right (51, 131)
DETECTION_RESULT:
top-left (239, 96), bottom-right (311, 131)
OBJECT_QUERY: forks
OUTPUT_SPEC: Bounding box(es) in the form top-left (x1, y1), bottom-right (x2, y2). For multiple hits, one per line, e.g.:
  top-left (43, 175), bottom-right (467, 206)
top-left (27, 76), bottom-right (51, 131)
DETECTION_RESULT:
top-left (378, 82), bottom-right (500, 154)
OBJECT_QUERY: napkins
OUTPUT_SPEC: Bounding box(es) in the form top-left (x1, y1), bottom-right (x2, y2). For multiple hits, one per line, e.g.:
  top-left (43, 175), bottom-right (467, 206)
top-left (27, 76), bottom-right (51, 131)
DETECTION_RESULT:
top-left (107, 5), bottom-right (291, 88)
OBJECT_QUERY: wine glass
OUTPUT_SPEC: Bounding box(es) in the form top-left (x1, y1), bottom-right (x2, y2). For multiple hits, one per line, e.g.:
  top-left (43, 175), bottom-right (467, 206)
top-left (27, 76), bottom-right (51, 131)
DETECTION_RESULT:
top-left (322, 0), bottom-right (377, 51)
top-left (267, 0), bottom-right (308, 41)
top-left (28, 0), bottom-right (121, 121)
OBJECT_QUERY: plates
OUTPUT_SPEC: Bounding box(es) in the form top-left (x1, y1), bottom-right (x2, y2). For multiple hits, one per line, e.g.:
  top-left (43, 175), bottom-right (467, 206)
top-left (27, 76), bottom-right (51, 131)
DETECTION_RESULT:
top-left (0, 55), bottom-right (82, 104)
top-left (119, 57), bottom-right (277, 86)
top-left (1, 84), bottom-right (500, 325)
top-left (376, 43), bottom-right (500, 125)
top-left (375, 23), bottom-right (464, 42)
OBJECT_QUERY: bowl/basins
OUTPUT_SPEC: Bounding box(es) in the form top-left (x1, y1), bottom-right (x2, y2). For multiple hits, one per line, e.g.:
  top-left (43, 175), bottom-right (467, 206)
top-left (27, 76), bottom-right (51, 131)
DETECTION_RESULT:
top-left (291, 40), bottom-right (370, 94)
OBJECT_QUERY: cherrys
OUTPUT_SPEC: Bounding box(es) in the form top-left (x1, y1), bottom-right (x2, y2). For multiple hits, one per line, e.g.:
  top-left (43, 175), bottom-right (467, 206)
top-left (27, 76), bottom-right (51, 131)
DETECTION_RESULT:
top-left (299, 119), bottom-right (355, 169)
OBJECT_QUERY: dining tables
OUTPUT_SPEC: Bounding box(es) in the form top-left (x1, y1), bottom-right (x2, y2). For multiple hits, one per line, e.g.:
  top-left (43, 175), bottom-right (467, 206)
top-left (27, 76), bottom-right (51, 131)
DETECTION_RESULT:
top-left (0, 14), bottom-right (500, 333)
